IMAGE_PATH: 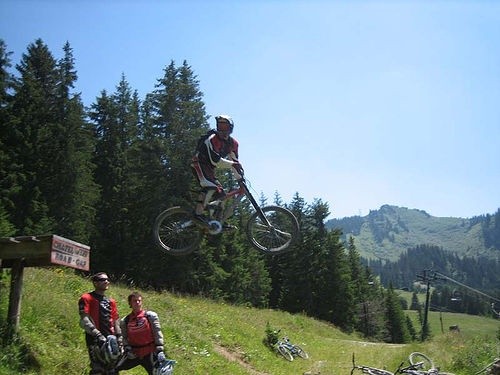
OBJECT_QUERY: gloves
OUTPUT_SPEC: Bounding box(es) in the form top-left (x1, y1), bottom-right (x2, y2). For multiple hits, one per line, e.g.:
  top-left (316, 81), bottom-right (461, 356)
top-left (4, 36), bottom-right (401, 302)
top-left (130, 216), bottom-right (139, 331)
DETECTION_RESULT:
top-left (97, 335), bottom-right (106, 347)
top-left (153, 360), bottom-right (165, 368)
top-left (125, 349), bottom-right (133, 359)
top-left (117, 346), bottom-right (124, 355)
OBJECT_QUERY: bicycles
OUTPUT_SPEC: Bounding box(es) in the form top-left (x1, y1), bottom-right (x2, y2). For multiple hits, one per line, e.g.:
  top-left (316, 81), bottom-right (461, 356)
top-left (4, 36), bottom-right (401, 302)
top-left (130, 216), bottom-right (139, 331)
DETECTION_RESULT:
top-left (278, 338), bottom-right (309, 361)
top-left (393, 352), bottom-right (455, 375)
top-left (153, 161), bottom-right (300, 256)
top-left (348, 351), bottom-right (396, 375)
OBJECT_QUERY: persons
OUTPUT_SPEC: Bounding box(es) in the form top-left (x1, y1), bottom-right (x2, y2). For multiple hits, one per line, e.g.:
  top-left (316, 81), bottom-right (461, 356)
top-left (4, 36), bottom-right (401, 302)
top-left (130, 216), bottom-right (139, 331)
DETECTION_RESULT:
top-left (115, 292), bottom-right (177, 375)
top-left (189, 114), bottom-right (246, 231)
top-left (78, 271), bottom-right (124, 375)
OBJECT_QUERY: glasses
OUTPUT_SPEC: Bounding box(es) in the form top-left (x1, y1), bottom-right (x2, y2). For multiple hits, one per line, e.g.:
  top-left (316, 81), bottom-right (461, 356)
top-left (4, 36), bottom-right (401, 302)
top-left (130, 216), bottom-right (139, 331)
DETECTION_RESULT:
top-left (97, 278), bottom-right (111, 282)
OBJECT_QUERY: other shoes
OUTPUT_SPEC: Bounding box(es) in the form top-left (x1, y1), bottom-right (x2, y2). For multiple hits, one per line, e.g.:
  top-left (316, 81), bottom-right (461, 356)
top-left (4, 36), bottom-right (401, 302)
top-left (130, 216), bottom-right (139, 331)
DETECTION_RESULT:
top-left (193, 213), bottom-right (209, 223)
top-left (221, 223), bottom-right (237, 232)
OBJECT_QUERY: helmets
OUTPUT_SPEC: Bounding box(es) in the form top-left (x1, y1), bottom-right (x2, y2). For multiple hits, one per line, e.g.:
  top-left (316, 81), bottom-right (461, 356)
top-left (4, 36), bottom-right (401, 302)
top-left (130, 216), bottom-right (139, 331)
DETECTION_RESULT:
top-left (213, 115), bottom-right (234, 135)
